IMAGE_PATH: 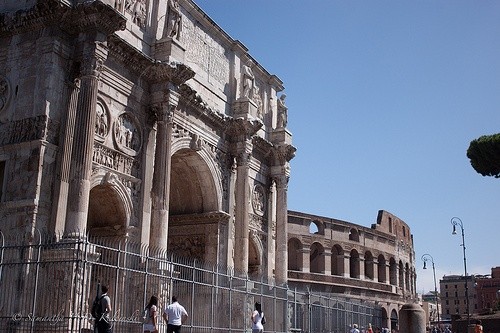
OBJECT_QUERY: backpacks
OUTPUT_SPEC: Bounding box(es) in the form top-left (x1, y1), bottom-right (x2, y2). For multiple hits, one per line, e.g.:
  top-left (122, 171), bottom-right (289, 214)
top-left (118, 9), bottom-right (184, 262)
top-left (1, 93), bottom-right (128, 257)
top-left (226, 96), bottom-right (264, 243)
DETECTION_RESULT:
top-left (91, 295), bottom-right (105, 318)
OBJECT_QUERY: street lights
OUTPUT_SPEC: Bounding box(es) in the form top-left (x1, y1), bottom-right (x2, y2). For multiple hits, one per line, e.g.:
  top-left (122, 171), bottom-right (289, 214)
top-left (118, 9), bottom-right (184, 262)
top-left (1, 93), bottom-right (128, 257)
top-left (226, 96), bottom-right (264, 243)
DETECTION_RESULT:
top-left (450, 216), bottom-right (470, 322)
top-left (421, 253), bottom-right (440, 326)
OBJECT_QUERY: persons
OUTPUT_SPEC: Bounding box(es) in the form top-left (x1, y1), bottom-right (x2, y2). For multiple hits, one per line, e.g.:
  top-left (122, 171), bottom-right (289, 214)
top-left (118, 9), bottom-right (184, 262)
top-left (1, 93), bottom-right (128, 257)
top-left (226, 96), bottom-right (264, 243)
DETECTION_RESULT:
top-left (432, 326), bottom-right (451, 333)
top-left (89, 282), bottom-right (113, 333)
top-left (240, 59), bottom-right (254, 99)
top-left (167, 0), bottom-right (181, 40)
top-left (115, 0), bottom-right (148, 28)
top-left (351, 324), bottom-right (360, 333)
top-left (143, 296), bottom-right (159, 333)
top-left (277, 93), bottom-right (288, 127)
top-left (251, 301), bottom-right (264, 333)
top-left (163, 296), bottom-right (188, 333)
top-left (475, 325), bottom-right (483, 333)
top-left (365, 323), bottom-right (390, 333)
